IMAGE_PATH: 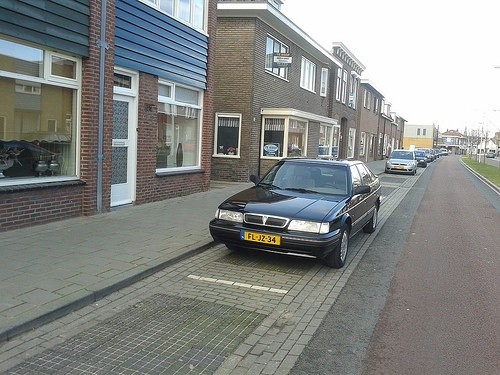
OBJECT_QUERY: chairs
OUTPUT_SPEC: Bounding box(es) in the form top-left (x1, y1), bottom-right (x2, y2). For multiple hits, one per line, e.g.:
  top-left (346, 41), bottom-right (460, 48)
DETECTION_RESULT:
top-left (292, 167), bottom-right (322, 188)
top-left (333, 171), bottom-right (346, 185)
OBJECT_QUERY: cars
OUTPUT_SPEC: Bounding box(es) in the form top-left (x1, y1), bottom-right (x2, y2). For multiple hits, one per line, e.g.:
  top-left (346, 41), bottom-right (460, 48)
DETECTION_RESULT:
top-left (384, 149), bottom-right (417, 175)
top-left (414, 147), bottom-right (449, 168)
top-left (209, 157), bottom-right (382, 268)
top-left (486, 152), bottom-right (495, 158)
